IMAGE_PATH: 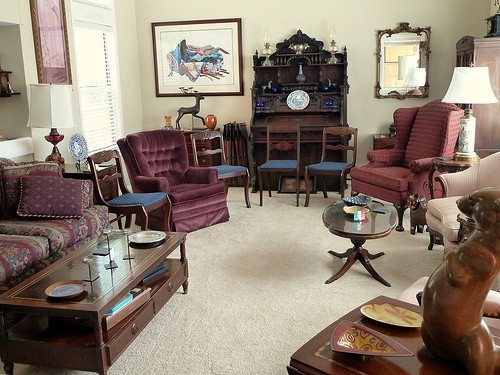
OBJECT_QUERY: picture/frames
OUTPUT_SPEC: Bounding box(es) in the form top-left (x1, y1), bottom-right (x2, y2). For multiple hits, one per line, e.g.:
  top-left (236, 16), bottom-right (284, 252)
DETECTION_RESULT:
top-left (151, 18), bottom-right (244, 97)
top-left (30, 0), bottom-right (73, 85)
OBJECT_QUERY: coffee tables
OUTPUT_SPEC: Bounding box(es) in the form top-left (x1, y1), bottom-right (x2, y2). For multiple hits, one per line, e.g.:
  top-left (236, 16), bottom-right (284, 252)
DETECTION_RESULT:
top-left (0, 230), bottom-right (188, 375)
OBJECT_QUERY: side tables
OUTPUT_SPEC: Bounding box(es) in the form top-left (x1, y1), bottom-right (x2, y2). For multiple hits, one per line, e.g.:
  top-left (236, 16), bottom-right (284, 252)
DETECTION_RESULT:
top-left (63, 164), bottom-right (123, 230)
top-left (428, 157), bottom-right (482, 250)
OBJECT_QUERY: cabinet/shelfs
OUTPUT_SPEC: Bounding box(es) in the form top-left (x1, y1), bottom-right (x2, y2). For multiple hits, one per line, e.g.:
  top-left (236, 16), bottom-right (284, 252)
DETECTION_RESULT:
top-left (456, 35), bottom-right (500, 151)
top-left (249, 29), bottom-right (350, 194)
top-left (186, 128), bottom-right (221, 167)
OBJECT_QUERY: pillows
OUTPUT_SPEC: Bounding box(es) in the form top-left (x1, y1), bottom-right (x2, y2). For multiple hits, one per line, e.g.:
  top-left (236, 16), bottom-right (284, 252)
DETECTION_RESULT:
top-left (16, 176), bottom-right (84, 219)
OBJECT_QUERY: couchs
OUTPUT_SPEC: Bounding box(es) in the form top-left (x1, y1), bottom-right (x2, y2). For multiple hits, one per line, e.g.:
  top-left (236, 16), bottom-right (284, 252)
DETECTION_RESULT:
top-left (116, 129), bottom-right (230, 233)
top-left (0, 158), bottom-right (113, 327)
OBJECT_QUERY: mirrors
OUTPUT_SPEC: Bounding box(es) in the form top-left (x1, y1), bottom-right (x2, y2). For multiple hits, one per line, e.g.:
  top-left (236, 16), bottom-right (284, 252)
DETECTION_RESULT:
top-left (376, 22), bottom-right (431, 100)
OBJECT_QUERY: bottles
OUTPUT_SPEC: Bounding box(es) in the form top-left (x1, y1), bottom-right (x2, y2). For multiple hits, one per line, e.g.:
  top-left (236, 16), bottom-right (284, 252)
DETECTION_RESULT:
top-left (297, 64), bottom-right (306, 82)
top-left (205, 115), bottom-right (217, 131)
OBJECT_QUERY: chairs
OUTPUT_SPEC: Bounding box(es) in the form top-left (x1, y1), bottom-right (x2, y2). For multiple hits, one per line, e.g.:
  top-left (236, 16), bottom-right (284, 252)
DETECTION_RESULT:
top-left (397, 276), bottom-right (500, 329)
top-left (190, 130), bottom-right (251, 209)
top-left (350, 98), bottom-right (464, 231)
top-left (87, 150), bottom-right (172, 232)
top-left (305, 127), bottom-right (358, 207)
top-left (257, 124), bottom-right (301, 207)
top-left (425, 151), bottom-right (500, 252)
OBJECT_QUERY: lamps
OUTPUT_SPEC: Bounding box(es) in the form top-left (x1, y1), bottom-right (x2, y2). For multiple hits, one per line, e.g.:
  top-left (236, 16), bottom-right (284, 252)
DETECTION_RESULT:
top-left (397, 55), bottom-right (427, 95)
top-left (441, 61), bottom-right (498, 161)
top-left (27, 84), bottom-right (74, 163)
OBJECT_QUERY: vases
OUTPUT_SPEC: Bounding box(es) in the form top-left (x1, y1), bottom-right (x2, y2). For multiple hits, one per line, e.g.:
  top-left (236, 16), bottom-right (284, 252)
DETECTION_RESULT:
top-left (296, 65), bottom-right (306, 83)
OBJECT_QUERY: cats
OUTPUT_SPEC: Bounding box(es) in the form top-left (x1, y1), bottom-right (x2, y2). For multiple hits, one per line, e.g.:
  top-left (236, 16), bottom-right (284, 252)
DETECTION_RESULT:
top-left (405, 191), bottom-right (428, 235)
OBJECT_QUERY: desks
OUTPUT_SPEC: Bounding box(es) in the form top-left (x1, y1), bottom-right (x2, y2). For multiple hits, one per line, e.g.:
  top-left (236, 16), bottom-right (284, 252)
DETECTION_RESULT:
top-left (286, 294), bottom-right (500, 374)
top-left (322, 200), bottom-right (398, 287)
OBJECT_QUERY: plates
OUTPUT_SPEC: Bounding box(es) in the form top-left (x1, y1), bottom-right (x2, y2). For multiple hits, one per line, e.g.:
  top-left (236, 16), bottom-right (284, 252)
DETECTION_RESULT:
top-left (341, 194), bottom-right (374, 205)
top-left (44, 279), bottom-right (87, 299)
top-left (130, 230), bottom-right (166, 244)
top-left (343, 206), bottom-right (370, 214)
top-left (287, 90), bottom-right (310, 110)
top-left (331, 320), bottom-right (415, 357)
top-left (69, 134), bottom-right (88, 160)
top-left (361, 303), bottom-right (423, 328)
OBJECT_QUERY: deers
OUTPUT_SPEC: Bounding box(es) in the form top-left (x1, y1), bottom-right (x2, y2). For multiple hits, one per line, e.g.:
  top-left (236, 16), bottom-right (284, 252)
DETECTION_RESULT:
top-left (175, 92), bottom-right (206, 130)
